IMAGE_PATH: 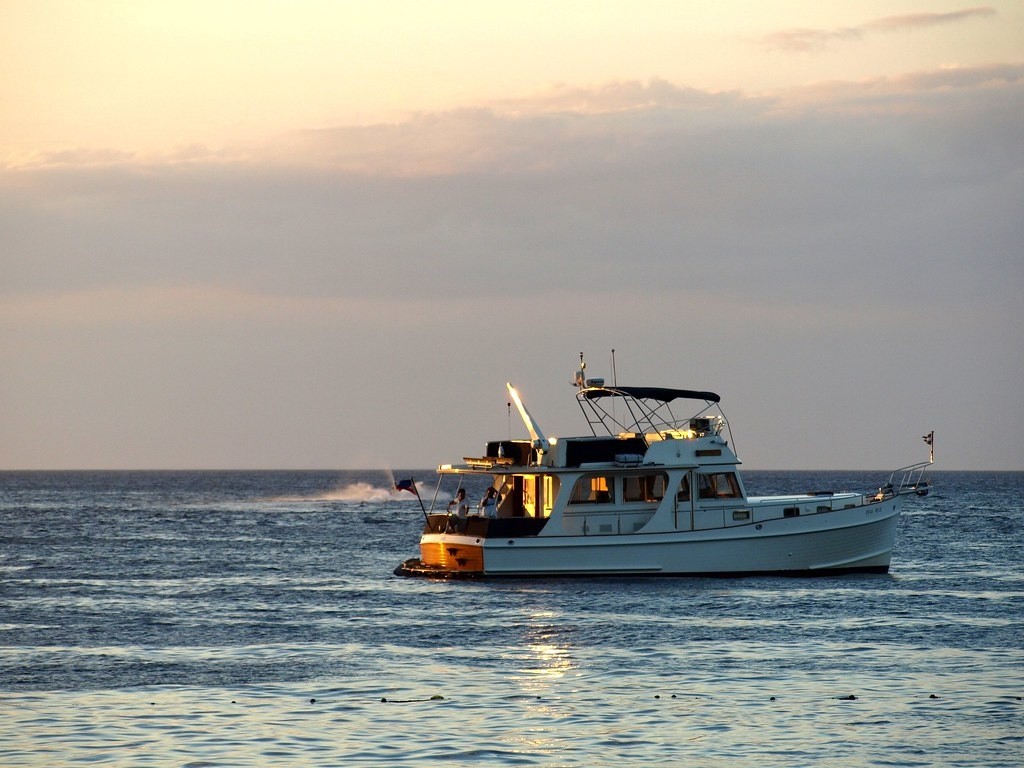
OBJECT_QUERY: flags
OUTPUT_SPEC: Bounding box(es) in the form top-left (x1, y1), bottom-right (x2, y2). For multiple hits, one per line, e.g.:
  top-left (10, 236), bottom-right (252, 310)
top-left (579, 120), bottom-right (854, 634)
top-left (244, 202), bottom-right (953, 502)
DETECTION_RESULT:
top-left (922, 433), bottom-right (932, 444)
top-left (395, 479), bottom-right (417, 495)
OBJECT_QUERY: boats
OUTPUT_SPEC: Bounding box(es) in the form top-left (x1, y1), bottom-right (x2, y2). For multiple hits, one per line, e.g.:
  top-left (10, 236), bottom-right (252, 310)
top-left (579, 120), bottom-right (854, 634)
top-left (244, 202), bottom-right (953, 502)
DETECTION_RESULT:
top-left (394, 347), bottom-right (937, 580)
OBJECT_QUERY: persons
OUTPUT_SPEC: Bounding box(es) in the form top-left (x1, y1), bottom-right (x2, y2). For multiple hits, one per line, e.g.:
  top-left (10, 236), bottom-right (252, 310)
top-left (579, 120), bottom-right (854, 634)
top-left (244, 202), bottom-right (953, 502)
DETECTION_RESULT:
top-left (447, 488), bottom-right (469, 535)
top-left (480, 487), bottom-right (502, 519)
